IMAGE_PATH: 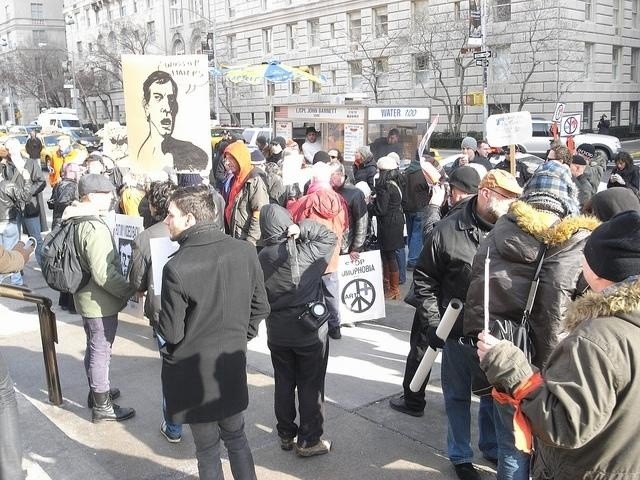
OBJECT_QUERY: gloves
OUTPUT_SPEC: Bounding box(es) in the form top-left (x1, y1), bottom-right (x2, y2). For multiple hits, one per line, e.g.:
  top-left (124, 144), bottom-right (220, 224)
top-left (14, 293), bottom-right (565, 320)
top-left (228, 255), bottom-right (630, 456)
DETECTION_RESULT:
top-left (425, 323), bottom-right (446, 351)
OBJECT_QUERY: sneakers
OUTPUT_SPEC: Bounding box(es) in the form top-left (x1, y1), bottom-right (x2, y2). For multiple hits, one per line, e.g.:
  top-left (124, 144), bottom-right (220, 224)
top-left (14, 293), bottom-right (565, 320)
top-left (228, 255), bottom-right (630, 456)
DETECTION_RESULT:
top-left (159, 418), bottom-right (182, 443)
top-left (406, 262), bottom-right (414, 271)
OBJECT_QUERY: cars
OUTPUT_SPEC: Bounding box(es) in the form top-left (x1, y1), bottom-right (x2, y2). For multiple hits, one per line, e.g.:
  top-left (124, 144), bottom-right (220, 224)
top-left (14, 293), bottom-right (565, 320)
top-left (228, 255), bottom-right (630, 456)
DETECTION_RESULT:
top-left (0, 125), bottom-right (103, 173)
top-left (211, 124), bottom-right (272, 151)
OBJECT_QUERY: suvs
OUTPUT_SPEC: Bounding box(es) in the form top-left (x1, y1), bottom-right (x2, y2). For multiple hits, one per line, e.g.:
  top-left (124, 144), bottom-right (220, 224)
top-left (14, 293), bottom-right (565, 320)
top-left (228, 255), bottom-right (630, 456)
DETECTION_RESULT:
top-left (515, 120), bottom-right (621, 164)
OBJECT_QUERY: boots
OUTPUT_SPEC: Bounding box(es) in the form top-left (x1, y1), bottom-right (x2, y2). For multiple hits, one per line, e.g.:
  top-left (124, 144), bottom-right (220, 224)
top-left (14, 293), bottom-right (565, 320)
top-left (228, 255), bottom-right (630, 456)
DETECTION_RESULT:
top-left (385, 270), bottom-right (400, 300)
top-left (91, 390), bottom-right (136, 424)
top-left (87, 378), bottom-right (120, 408)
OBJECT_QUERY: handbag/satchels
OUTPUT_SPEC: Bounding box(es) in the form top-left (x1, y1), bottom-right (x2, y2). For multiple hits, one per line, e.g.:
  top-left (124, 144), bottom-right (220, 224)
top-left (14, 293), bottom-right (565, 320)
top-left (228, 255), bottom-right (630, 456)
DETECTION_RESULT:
top-left (457, 319), bottom-right (535, 398)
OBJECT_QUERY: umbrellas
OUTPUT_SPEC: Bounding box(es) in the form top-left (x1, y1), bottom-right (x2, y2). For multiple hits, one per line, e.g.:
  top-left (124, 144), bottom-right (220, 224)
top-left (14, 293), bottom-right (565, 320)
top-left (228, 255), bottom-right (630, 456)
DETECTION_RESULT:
top-left (225, 58), bottom-right (323, 140)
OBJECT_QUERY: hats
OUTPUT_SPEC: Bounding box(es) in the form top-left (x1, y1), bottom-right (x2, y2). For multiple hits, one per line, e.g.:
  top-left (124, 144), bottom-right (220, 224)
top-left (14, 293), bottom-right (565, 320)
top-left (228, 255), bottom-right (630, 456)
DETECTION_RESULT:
top-left (313, 151), bottom-right (330, 163)
top-left (583, 210), bottom-right (640, 282)
top-left (78, 174), bottom-right (114, 196)
top-left (271, 136), bottom-right (286, 149)
top-left (590, 187), bottom-right (639, 220)
top-left (358, 145), bottom-right (400, 170)
top-left (448, 138), bottom-right (596, 194)
top-left (306, 126), bottom-right (316, 135)
top-left (85, 154), bottom-right (105, 166)
top-left (251, 150), bottom-right (266, 165)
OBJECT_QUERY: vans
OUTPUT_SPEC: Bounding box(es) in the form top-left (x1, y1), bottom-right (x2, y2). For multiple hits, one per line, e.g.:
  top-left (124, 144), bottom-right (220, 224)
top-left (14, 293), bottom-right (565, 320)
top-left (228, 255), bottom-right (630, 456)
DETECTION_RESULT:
top-left (37, 114), bottom-right (81, 133)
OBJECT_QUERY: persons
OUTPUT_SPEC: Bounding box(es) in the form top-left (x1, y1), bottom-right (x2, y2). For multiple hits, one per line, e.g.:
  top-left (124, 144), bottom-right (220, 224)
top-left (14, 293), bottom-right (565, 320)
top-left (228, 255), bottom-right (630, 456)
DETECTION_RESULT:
top-left (137, 71), bottom-right (208, 170)
top-left (0, 127), bottom-right (137, 424)
top-left (127, 115), bottom-right (640, 480)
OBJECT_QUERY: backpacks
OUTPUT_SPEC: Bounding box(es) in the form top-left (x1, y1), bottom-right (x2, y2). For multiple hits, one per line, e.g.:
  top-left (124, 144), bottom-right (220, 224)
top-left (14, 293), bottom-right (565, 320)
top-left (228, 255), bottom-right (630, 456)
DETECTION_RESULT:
top-left (39, 216), bottom-right (101, 295)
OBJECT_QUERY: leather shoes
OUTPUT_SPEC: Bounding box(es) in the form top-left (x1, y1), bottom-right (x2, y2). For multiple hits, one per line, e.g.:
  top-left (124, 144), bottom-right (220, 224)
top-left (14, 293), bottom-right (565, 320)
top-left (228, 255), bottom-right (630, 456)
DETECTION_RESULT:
top-left (455, 460), bottom-right (481, 480)
top-left (390, 397), bottom-right (424, 417)
top-left (295, 438), bottom-right (333, 457)
top-left (329, 326), bottom-right (342, 339)
top-left (280, 436), bottom-right (294, 450)
